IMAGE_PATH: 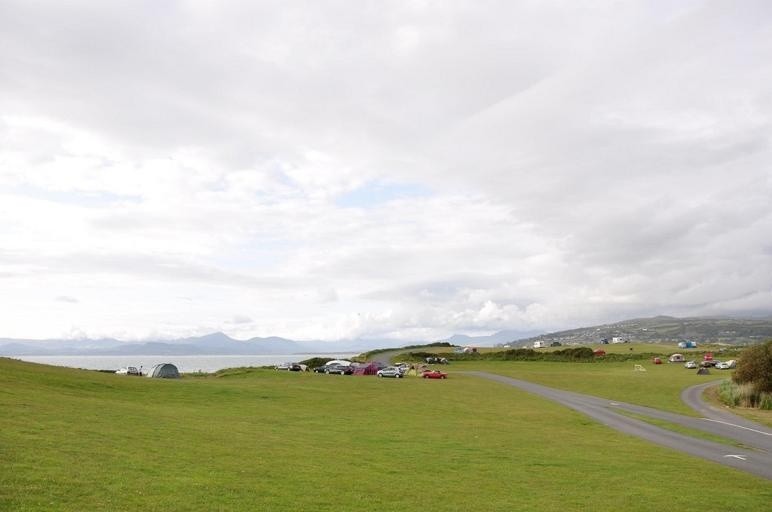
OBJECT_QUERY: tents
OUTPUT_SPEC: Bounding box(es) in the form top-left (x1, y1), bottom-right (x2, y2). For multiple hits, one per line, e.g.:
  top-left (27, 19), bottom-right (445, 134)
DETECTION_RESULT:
top-left (146, 363), bottom-right (180, 378)
top-left (696, 367), bottom-right (711, 375)
top-left (403, 364), bottom-right (432, 377)
top-left (354, 361), bottom-right (386, 375)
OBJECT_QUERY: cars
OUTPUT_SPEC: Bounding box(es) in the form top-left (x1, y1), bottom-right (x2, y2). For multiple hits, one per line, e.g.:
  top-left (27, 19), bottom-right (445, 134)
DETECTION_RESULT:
top-left (720, 348), bottom-right (729, 353)
top-left (653, 357), bottom-right (662, 364)
top-left (504, 344), bottom-right (511, 348)
top-left (521, 345), bottom-right (528, 349)
top-left (600, 339), bottom-right (608, 345)
top-left (114, 366), bottom-right (138, 376)
top-left (594, 349), bottom-right (605, 356)
top-left (273, 358), bottom-right (448, 379)
top-left (683, 358), bottom-right (729, 370)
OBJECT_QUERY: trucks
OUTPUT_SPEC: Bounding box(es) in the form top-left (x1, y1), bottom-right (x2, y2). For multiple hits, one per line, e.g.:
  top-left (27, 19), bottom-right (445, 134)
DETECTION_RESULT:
top-left (612, 336), bottom-right (628, 344)
top-left (534, 341), bottom-right (546, 348)
top-left (454, 345), bottom-right (480, 354)
